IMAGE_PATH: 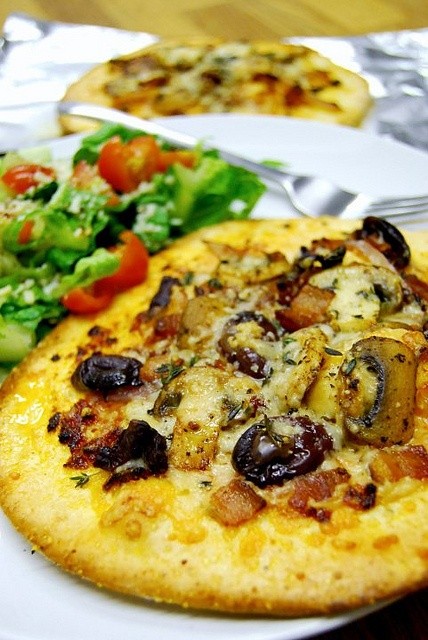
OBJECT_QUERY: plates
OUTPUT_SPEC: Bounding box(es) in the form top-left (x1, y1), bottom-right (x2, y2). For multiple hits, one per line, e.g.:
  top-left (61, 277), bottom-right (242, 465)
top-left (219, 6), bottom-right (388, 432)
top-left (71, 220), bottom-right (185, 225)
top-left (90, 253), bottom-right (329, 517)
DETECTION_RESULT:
top-left (0, 115), bottom-right (428, 640)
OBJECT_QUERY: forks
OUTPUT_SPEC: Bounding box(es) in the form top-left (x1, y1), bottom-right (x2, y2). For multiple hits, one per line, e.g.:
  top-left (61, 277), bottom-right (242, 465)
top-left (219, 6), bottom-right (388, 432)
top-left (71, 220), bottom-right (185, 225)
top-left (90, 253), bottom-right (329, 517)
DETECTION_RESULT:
top-left (61, 104), bottom-right (427, 222)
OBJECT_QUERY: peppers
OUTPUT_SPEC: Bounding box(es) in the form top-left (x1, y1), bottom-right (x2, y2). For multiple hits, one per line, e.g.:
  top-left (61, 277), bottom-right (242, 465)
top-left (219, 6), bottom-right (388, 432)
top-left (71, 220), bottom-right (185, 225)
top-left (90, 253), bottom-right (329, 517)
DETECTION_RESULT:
top-left (0, 124), bottom-right (267, 383)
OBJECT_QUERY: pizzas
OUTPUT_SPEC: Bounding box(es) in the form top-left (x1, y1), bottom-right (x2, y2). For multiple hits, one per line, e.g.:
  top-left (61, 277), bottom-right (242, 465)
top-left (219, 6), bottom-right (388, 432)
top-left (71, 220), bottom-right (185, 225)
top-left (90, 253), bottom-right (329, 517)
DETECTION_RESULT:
top-left (0, 217), bottom-right (427, 618)
top-left (58, 35), bottom-right (372, 139)
